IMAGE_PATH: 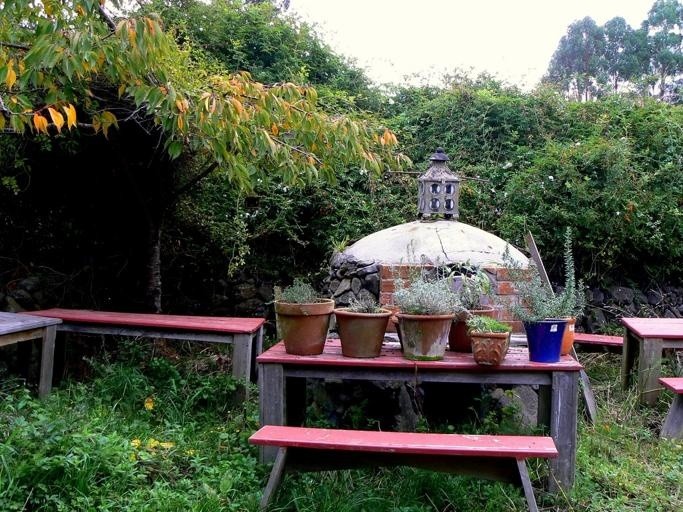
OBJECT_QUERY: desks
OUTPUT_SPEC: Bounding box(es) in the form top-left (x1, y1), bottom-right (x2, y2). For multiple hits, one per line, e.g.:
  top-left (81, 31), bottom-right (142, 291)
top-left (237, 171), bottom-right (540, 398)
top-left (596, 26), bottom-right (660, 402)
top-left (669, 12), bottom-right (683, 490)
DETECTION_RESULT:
top-left (15, 308), bottom-right (266, 399)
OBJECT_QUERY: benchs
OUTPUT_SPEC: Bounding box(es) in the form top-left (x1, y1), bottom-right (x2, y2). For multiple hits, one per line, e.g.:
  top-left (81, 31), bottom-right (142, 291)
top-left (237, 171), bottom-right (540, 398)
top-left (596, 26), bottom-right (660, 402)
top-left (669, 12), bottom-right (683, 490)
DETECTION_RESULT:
top-left (574, 317), bottom-right (682, 441)
top-left (248, 425), bottom-right (558, 512)
